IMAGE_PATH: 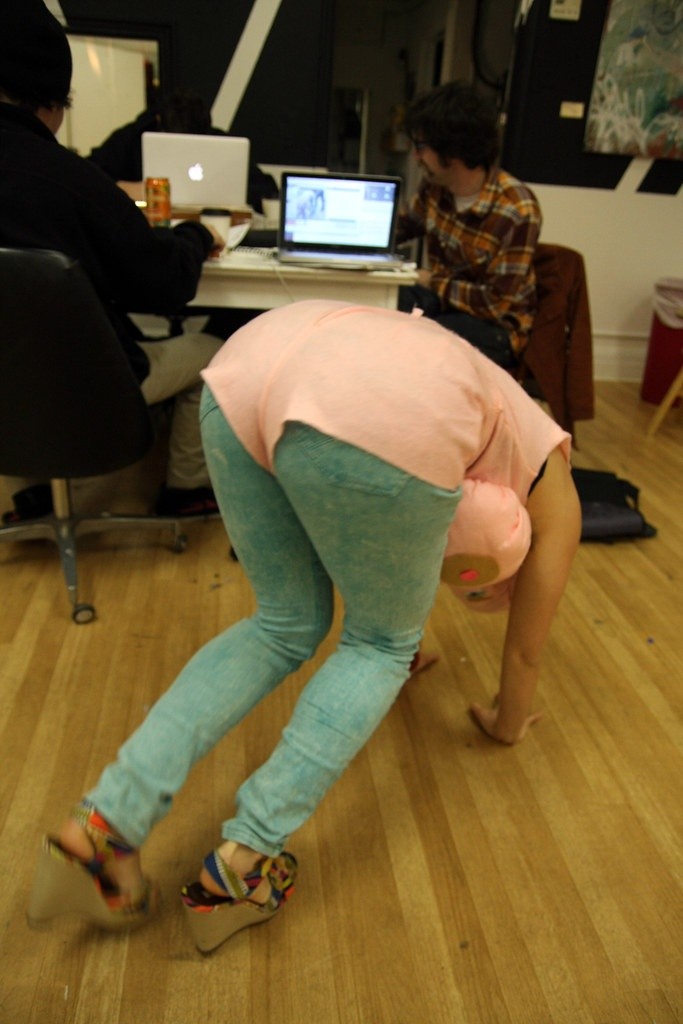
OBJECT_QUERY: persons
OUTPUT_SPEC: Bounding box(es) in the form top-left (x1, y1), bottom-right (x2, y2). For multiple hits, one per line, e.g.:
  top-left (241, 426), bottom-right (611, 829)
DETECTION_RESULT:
top-left (1, 0), bottom-right (229, 522)
top-left (88, 75), bottom-right (282, 213)
top-left (28, 299), bottom-right (584, 954)
top-left (388, 82), bottom-right (543, 379)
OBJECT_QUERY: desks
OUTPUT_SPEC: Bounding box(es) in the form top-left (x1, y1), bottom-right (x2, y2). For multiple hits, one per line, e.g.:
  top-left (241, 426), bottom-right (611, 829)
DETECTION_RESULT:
top-left (196, 247), bottom-right (420, 311)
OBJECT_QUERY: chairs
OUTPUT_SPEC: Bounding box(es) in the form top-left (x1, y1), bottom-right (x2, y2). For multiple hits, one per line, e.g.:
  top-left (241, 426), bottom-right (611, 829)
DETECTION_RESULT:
top-left (0, 246), bottom-right (191, 623)
top-left (518, 241), bottom-right (578, 429)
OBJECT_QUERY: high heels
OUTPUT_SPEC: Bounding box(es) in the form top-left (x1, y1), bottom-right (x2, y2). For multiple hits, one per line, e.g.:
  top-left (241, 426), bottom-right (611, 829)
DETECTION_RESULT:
top-left (179, 844), bottom-right (298, 955)
top-left (25, 814), bottom-right (162, 931)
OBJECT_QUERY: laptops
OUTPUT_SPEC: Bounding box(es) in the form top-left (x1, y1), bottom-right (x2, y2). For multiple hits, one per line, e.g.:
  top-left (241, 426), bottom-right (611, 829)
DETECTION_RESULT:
top-left (276, 171), bottom-right (404, 268)
top-left (142, 133), bottom-right (249, 208)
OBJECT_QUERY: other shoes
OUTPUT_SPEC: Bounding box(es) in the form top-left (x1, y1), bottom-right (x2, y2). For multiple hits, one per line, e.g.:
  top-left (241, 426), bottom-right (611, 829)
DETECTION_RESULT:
top-left (144, 479), bottom-right (220, 522)
top-left (2, 482), bottom-right (56, 521)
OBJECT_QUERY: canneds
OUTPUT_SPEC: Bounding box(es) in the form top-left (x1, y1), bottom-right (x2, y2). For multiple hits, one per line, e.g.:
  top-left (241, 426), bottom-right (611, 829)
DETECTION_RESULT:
top-left (146, 177), bottom-right (170, 227)
